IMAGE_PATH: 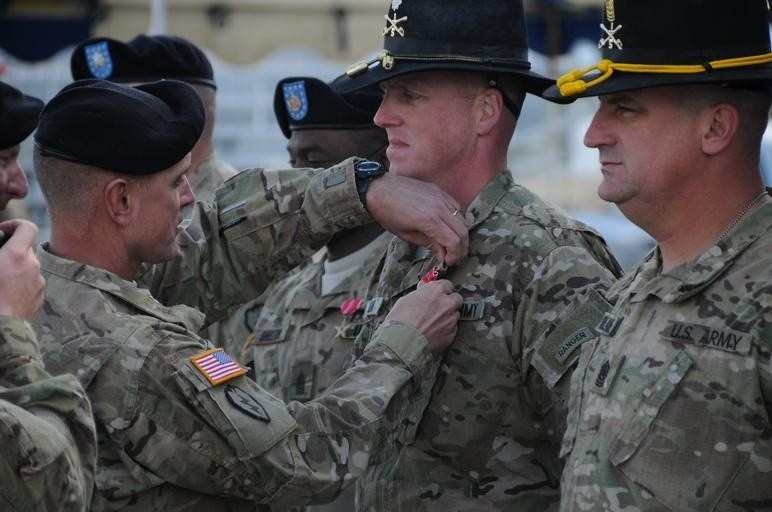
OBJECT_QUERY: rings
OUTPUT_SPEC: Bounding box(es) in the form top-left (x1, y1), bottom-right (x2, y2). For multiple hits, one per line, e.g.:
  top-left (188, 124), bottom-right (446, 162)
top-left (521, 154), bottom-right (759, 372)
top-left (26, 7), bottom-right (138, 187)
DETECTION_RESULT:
top-left (453, 210), bottom-right (458, 216)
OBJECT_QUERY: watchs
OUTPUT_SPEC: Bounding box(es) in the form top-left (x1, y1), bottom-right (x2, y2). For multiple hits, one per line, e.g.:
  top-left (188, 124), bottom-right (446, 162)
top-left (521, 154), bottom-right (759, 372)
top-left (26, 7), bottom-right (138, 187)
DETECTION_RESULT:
top-left (352, 160), bottom-right (388, 209)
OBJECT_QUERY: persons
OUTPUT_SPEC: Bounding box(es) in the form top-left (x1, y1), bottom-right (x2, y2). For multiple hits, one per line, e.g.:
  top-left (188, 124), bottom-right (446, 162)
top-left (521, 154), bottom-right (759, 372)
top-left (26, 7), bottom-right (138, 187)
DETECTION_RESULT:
top-left (0, 218), bottom-right (97, 512)
top-left (2, 78), bottom-right (42, 218)
top-left (236, 54), bottom-right (397, 511)
top-left (31, 78), bottom-right (471, 512)
top-left (544, 1), bottom-right (771, 512)
top-left (74, 32), bottom-right (292, 390)
top-left (347, 0), bottom-right (622, 512)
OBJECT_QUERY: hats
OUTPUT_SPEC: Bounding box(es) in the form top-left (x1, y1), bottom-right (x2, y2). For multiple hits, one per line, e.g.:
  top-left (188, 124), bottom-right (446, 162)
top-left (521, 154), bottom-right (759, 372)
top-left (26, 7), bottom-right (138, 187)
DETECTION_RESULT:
top-left (273, 76), bottom-right (383, 140)
top-left (330, 0), bottom-right (577, 106)
top-left (0, 80), bottom-right (46, 149)
top-left (544, 0), bottom-right (771, 99)
top-left (70, 32), bottom-right (218, 90)
top-left (34, 78), bottom-right (206, 176)
